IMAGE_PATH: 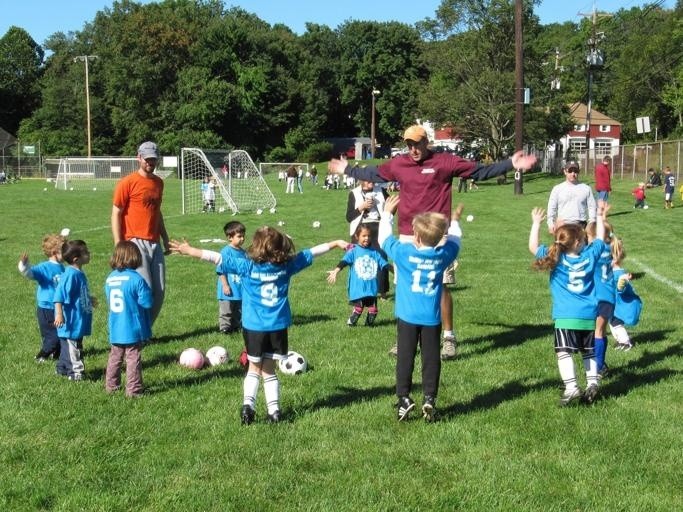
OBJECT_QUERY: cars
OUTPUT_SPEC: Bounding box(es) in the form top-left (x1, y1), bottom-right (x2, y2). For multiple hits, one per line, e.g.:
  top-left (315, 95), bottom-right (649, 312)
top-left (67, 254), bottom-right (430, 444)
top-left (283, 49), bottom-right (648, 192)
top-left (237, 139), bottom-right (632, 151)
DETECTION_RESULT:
top-left (391, 146), bottom-right (409, 158)
top-left (611, 155), bottom-right (638, 173)
top-left (339, 146), bottom-right (355, 157)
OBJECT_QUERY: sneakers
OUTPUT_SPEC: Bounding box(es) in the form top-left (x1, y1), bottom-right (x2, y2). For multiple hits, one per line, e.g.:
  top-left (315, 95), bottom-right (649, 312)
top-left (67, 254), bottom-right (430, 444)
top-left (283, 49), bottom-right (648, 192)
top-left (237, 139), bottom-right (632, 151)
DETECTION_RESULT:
top-left (441, 334), bottom-right (460, 360)
top-left (611, 342), bottom-right (623, 350)
top-left (596, 363), bottom-right (609, 380)
top-left (622, 341), bottom-right (632, 352)
top-left (220, 328), bottom-right (229, 334)
top-left (579, 383), bottom-right (598, 405)
top-left (240, 405), bottom-right (255, 426)
top-left (421, 395), bottom-right (437, 426)
top-left (557, 387), bottom-right (583, 407)
top-left (389, 344), bottom-right (398, 357)
top-left (397, 397), bottom-right (416, 423)
top-left (265, 411), bottom-right (281, 424)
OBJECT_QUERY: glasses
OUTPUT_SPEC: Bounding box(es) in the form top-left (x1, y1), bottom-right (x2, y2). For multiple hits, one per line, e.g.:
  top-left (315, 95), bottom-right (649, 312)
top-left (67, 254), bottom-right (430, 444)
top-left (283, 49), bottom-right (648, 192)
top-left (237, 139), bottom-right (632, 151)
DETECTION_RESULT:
top-left (567, 169), bottom-right (578, 174)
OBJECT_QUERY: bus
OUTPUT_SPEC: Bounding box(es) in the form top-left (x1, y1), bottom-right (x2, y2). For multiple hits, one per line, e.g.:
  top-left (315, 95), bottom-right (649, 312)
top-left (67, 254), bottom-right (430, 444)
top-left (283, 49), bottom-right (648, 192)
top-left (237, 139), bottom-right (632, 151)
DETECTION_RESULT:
top-left (44, 159), bottom-right (99, 178)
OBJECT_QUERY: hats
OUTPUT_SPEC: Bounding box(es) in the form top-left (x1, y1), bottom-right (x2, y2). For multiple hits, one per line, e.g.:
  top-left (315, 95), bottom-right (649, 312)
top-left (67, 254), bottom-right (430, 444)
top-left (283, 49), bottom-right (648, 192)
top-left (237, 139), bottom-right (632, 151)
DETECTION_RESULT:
top-left (138, 140), bottom-right (159, 160)
top-left (403, 125), bottom-right (427, 143)
top-left (565, 160), bottom-right (580, 169)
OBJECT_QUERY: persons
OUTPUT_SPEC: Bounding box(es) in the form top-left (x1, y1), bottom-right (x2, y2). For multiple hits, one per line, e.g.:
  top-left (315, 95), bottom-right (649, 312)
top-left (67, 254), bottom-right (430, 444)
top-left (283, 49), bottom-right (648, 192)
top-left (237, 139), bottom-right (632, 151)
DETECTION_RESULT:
top-left (296, 166), bottom-right (303, 194)
top-left (528, 198), bottom-right (611, 407)
top-left (202, 175), bottom-right (224, 212)
top-left (548, 159), bottom-right (596, 244)
top-left (378, 194), bottom-right (464, 421)
top-left (662, 166), bottom-right (676, 209)
top-left (342, 172), bottom-right (359, 190)
top-left (346, 179), bottom-right (400, 302)
top-left (104, 240), bottom-right (155, 397)
top-left (52, 239), bottom-right (100, 382)
top-left (285, 164), bottom-right (297, 193)
top-left (110, 142), bottom-right (172, 347)
top-left (469, 179), bottom-right (479, 190)
top-left (632, 182), bottom-right (649, 209)
top-left (325, 172), bottom-right (333, 190)
top-left (645, 169), bottom-right (661, 188)
top-left (334, 172), bottom-right (340, 189)
top-left (608, 237), bottom-right (643, 352)
top-left (284, 169), bottom-right (288, 181)
top-left (18, 235), bottom-right (67, 364)
top-left (326, 225), bottom-right (395, 328)
top-left (200, 174), bottom-right (210, 203)
top-left (594, 156), bottom-right (612, 199)
top-left (582, 201), bottom-right (617, 381)
top-left (279, 169), bottom-right (283, 182)
top-left (311, 165), bottom-right (318, 185)
top-left (216, 221), bottom-right (251, 335)
top-left (457, 176), bottom-right (467, 193)
top-left (167, 226), bottom-right (357, 425)
top-left (327, 123), bottom-right (538, 361)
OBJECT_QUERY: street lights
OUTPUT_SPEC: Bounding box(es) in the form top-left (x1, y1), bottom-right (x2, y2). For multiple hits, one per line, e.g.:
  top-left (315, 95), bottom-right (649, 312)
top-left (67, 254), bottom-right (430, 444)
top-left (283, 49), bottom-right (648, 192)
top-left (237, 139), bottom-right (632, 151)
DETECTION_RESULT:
top-left (72, 54), bottom-right (98, 157)
top-left (370, 90), bottom-right (379, 159)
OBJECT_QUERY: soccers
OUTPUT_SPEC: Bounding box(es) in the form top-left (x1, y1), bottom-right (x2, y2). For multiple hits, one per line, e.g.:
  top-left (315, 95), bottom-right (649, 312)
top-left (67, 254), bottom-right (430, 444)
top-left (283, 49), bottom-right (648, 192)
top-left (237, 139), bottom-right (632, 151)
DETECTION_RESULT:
top-left (279, 351), bottom-right (306, 375)
top-left (180, 348), bottom-right (204, 370)
top-left (204, 346), bottom-right (229, 368)
